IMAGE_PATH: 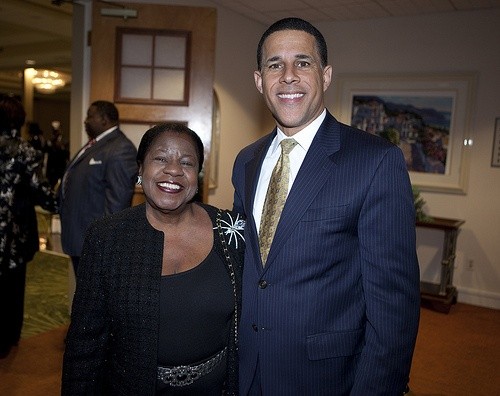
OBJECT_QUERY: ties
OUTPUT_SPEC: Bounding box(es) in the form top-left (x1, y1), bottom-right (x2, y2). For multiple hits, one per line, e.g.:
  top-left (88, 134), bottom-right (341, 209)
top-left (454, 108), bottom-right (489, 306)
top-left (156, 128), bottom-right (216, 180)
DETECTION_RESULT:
top-left (259, 138), bottom-right (298, 269)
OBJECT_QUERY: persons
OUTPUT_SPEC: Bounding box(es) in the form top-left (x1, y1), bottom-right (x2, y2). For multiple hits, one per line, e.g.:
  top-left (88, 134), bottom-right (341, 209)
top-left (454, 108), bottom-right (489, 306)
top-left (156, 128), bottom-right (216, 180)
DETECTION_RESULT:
top-left (57, 100), bottom-right (140, 277)
top-left (61, 120), bottom-right (244, 396)
top-left (232, 17), bottom-right (422, 396)
top-left (0, 91), bottom-right (68, 358)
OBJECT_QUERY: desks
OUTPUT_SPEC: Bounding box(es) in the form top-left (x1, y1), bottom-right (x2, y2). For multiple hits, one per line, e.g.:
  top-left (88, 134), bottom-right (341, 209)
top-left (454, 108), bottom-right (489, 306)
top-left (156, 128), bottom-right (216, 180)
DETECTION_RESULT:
top-left (416, 216), bottom-right (466, 313)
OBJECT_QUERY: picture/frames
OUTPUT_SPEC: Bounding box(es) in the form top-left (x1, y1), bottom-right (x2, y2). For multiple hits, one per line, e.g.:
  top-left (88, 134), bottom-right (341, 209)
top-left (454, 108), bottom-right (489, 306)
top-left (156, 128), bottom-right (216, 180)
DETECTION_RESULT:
top-left (332, 72), bottom-right (478, 194)
top-left (491, 118), bottom-right (500, 169)
